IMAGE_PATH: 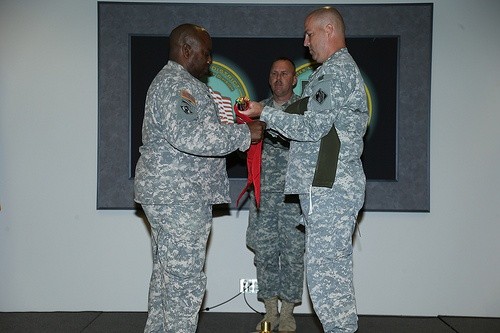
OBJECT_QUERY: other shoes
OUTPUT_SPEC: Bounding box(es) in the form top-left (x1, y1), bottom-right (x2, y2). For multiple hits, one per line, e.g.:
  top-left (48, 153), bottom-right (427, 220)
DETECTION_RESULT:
top-left (278, 299), bottom-right (296, 332)
top-left (256, 296), bottom-right (279, 333)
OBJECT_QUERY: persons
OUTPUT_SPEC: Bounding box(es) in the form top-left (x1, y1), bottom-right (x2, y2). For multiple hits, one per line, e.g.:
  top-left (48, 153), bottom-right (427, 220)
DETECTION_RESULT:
top-left (233, 6), bottom-right (371, 333)
top-left (132, 23), bottom-right (267, 332)
top-left (245, 57), bottom-right (308, 332)
